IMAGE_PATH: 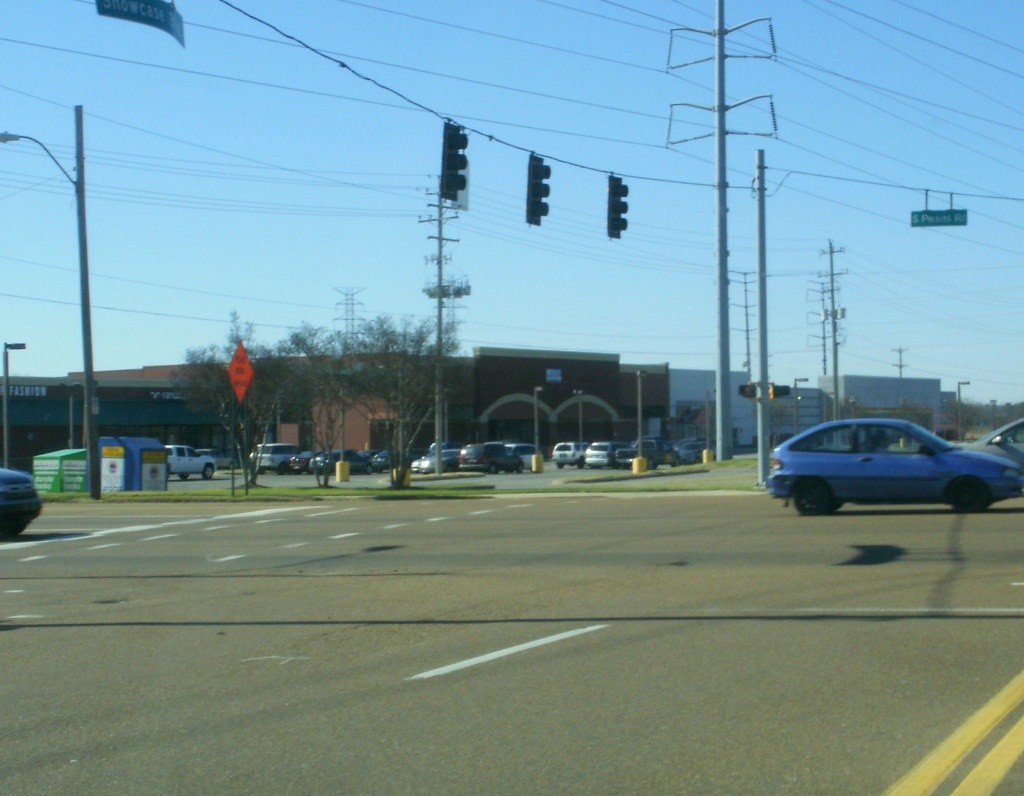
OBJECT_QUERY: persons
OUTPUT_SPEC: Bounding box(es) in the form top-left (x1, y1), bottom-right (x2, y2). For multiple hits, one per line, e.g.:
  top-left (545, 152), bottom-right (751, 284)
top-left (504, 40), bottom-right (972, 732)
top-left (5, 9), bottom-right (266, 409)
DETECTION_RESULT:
top-left (872, 429), bottom-right (891, 452)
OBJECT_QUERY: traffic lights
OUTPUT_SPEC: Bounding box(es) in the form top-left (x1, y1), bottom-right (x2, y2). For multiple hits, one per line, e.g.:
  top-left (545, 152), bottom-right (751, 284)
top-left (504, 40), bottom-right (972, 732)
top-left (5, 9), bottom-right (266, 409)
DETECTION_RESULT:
top-left (771, 384), bottom-right (791, 399)
top-left (607, 174), bottom-right (628, 239)
top-left (526, 154), bottom-right (550, 226)
top-left (439, 122), bottom-right (468, 202)
top-left (738, 385), bottom-right (756, 397)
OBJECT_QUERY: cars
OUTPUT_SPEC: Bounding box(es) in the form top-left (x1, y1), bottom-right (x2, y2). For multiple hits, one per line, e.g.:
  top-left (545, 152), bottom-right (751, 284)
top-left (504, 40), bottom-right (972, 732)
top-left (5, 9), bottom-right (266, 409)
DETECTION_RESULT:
top-left (290, 450), bottom-right (325, 474)
top-left (195, 449), bottom-right (238, 470)
top-left (309, 449), bottom-right (374, 475)
top-left (357, 450), bottom-right (460, 474)
top-left (764, 418), bottom-right (1023, 515)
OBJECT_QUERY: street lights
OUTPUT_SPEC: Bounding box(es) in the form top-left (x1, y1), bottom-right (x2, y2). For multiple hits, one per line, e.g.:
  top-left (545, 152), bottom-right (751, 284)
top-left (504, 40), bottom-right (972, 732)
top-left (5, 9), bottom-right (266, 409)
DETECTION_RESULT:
top-left (378, 365), bottom-right (411, 488)
top-left (632, 370), bottom-right (648, 473)
top-left (573, 390), bottom-right (584, 452)
top-left (794, 378), bottom-right (808, 436)
top-left (959, 382), bottom-right (970, 440)
top-left (531, 386), bottom-right (545, 473)
top-left (3, 343), bottom-right (26, 469)
top-left (702, 388), bottom-right (716, 463)
top-left (0, 104), bottom-right (101, 499)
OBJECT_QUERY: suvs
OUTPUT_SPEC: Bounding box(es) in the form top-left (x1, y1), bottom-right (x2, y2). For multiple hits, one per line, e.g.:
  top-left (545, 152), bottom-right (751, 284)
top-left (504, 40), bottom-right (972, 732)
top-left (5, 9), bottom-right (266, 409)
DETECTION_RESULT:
top-left (250, 443), bottom-right (301, 475)
top-left (0, 468), bottom-right (42, 537)
top-left (164, 445), bottom-right (216, 480)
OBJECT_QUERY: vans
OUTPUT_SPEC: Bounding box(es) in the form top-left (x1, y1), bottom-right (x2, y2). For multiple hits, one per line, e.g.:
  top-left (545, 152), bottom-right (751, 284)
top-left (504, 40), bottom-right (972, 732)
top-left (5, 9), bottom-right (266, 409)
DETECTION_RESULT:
top-left (430, 436), bottom-right (713, 474)
